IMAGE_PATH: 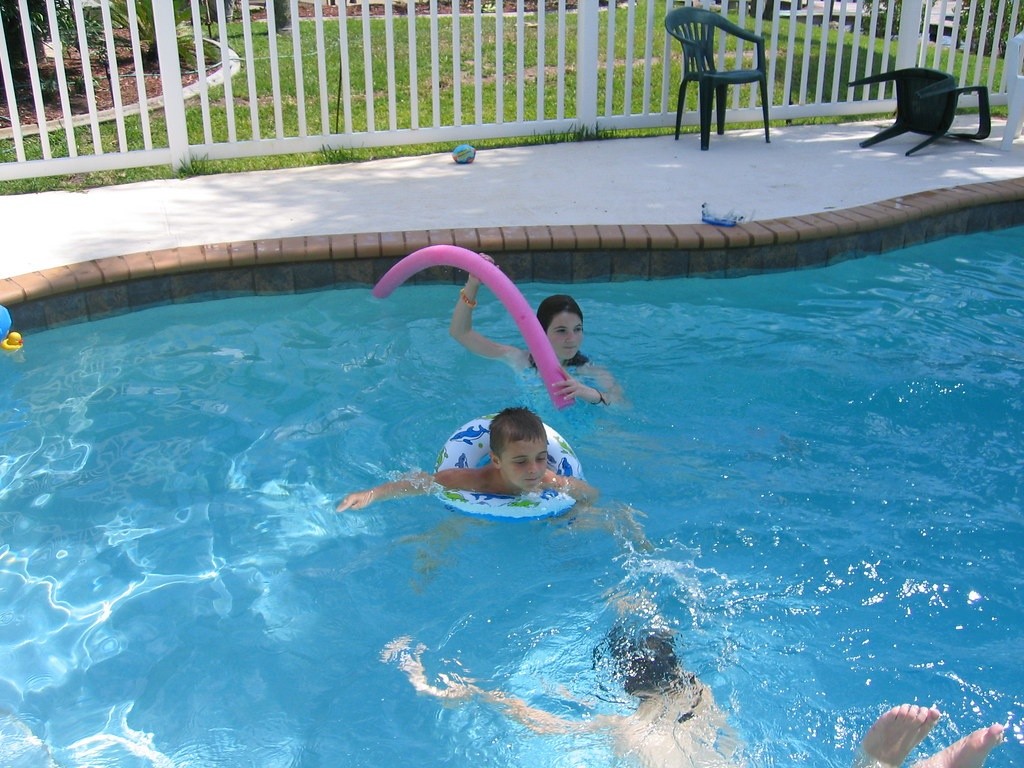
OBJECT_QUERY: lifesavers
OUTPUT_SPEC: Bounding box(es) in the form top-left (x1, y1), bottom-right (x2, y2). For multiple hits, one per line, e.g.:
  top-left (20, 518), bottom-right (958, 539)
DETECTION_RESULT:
top-left (431, 414), bottom-right (586, 523)
top-left (372, 243), bottom-right (575, 409)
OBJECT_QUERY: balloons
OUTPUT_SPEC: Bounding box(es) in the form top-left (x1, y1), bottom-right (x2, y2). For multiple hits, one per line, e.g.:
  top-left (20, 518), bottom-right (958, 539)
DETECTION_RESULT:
top-left (371, 244), bottom-right (574, 408)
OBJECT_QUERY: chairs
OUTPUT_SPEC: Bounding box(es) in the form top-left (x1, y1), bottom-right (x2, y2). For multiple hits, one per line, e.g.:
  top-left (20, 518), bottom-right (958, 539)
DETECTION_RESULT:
top-left (664, 7), bottom-right (771, 153)
top-left (998, 30), bottom-right (1024, 150)
top-left (845, 67), bottom-right (991, 156)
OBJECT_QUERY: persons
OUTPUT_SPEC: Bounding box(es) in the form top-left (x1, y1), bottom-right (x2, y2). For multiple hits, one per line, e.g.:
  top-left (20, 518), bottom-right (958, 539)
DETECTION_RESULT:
top-left (866, 701), bottom-right (1006, 767)
top-left (336, 408), bottom-right (560, 513)
top-left (449, 252), bottom-right (615, 406)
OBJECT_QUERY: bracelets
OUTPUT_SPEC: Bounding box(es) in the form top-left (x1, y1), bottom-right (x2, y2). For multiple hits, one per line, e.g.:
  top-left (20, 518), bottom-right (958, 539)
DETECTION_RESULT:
top-left (590, 387), bottom-right (610, 406)
top-left (462, 288), bottom-right (476, 307)
